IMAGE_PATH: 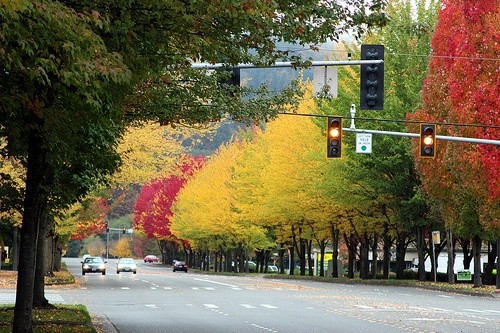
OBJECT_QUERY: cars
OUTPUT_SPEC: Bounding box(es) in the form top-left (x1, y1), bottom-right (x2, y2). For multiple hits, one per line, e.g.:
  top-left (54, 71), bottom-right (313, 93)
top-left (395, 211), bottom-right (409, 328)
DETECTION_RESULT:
top-left (173, 261), bottom-right (188, 273)
top-left (81, 254), bottom-right (107, 275)
top-left (116, 257), bottom-right (137, 274)
top-left (268, 266), bottom-right (278, 273)
top-left (144, 255), bottom-right (159, 263)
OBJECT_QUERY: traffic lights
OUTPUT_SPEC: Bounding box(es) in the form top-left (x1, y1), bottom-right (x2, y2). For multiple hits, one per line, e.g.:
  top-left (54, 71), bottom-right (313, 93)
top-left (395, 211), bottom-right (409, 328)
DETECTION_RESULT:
top-left (123, 228), bottom-right (125, 234)
top-left (106, 227), bottom-right (109, 232)
top-left (327, 117), bottom-right (342, 158)
top-left (419, 123), bottom-right (437, 159)
